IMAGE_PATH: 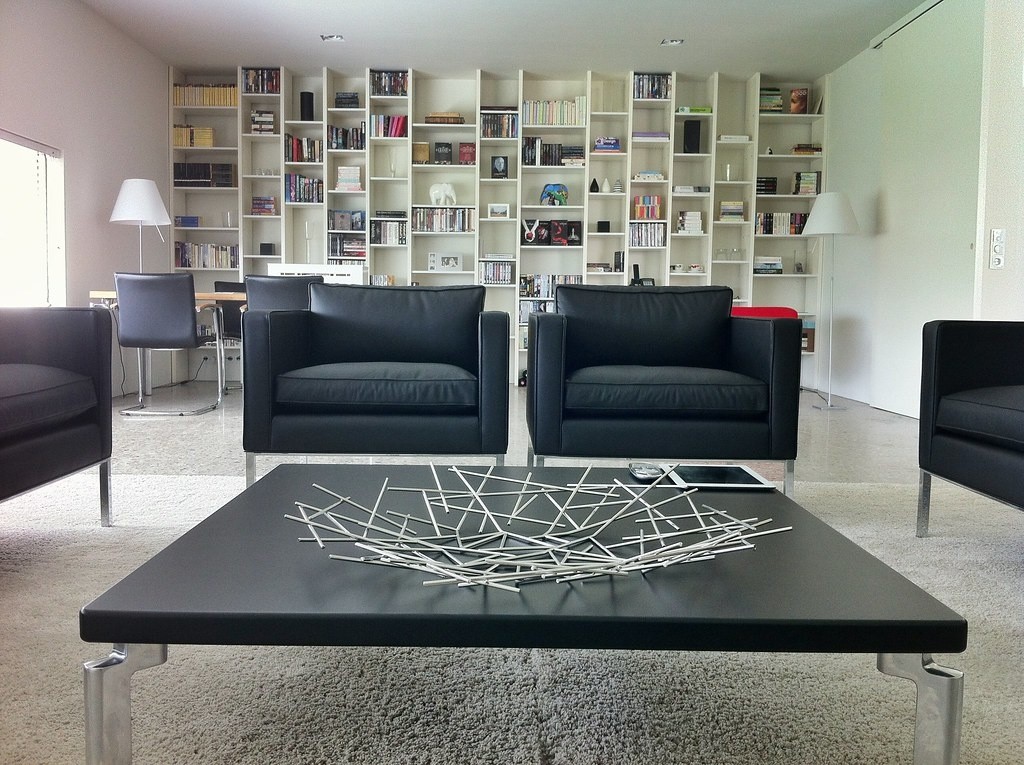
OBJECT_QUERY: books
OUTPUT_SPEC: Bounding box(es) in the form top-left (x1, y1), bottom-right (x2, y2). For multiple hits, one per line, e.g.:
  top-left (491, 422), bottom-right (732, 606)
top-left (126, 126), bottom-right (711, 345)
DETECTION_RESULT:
top-left (284, 134), bottom-right (323, 162)
top-left (754, 86), bottom-right (823, 353)
top-left (242, 68), bottom-right (280, 94)
top-left (719, 134), bottom-right (751, 141)
top-left (478, 219), bottom-right (623, 349)
top-left (369, 71), bottom-right (476, 166)
top-left (479, 73), bottom-right (671, 179)
top-left (629, 171), bottom-right (746, 247)
top-left (250, 109), bottom-right (275, 134)
top-left (675, 106), bottom-right (713, 112)
top-left (335, 91), bottom-right (359, 108)
top-left (327, 209), bottom-right (367, 268)
top-left (370, 274), bottom-right (395, 286)
top-left (197, 325), bottom-right (241, 347)
top-left (174, 83), bottom-right (239, 268)
top-left (285, 172), bottom-right (322, 202)
top-left (327, 121), bottom-right (365, 149)
top-left (370, 207), bottom-right (473, 246)
top-left (251, 196), bottom-right (279, 216)
top-left (334, 166), bottom-right (363, 190)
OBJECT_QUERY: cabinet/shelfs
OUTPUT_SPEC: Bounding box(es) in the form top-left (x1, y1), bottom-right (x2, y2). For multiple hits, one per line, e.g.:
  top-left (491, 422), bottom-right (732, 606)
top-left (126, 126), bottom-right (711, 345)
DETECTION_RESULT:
top-left (164, 59), bottom-right (826, 357)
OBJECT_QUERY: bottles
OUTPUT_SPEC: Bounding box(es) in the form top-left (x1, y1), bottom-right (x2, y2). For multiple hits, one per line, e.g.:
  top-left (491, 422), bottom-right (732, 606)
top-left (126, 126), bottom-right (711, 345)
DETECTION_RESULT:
top-left (730, 249), bottom-right (741, 261)
top-left (590, 178), bottom-right (599, 192)
top-left (613, 178), bottom-right (622, 193)
top-left (602, 178), bottom-right (610, 193)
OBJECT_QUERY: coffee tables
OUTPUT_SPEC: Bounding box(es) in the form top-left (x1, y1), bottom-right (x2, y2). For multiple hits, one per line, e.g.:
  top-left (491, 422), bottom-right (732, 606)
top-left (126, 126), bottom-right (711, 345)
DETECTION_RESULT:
top-left (78, 462), bottom-right (967, 764)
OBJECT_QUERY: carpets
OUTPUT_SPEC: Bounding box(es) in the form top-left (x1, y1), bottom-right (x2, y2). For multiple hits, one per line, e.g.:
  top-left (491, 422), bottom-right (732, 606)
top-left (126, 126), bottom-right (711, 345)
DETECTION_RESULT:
top-left (0, 476), bottom-right (1023, 765)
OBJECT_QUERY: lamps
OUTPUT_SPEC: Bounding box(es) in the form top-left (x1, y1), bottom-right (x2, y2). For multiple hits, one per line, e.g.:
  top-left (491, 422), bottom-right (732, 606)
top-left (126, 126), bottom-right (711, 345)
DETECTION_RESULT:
top-left (801, 190), bottom-right (861, 409)
top-left (109, 177), bottom-right (171, 273)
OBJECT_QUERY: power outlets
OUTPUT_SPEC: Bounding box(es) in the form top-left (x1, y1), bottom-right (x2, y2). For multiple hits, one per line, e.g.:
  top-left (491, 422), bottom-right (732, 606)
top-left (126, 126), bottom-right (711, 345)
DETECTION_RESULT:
top-left (227, 355), bottom-right (241, 362)
top-left (201, 354), bottom-right (217, 363)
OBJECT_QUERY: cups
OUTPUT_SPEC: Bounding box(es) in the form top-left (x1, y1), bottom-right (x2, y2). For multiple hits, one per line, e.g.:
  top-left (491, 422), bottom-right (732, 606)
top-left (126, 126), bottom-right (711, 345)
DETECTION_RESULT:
top-left (714, 248), bottom-right (728, 260)
top-left (222, 210), bottom-right (238, 227)
top-left (670, 264), bottom-right (683, 270)
top-left (690, 264), bottom-right (702, 270)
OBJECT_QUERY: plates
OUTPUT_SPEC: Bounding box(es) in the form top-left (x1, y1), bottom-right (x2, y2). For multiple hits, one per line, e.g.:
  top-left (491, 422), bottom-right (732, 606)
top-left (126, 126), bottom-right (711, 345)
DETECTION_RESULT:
top-left (688, 270), bottom-right (703, 273)
top-left (673, 270), bottom-right (687, 273)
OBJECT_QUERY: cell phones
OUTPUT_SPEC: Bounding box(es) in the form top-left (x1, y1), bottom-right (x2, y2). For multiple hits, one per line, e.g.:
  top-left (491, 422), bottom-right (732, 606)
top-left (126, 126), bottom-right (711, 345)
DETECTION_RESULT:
top-left (629, 463), bottom-right (661, 480)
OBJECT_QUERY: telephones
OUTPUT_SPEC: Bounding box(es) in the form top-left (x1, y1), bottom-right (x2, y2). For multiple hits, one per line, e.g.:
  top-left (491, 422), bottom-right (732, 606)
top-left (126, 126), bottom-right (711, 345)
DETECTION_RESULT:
top-left (631, 264), bottom-right (655, 286)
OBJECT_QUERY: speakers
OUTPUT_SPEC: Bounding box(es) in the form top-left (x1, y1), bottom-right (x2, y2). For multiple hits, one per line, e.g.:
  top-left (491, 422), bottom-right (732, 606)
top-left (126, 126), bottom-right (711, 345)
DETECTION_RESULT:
top-left (300, 92), bottom-right (314, 121)
top-left (684, 120), bottom-right (700, 154)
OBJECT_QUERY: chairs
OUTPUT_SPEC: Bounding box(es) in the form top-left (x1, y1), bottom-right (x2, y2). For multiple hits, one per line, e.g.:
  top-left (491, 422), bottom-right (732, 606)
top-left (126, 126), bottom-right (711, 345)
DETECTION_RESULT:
top-left (239, 273), bottom-right (323, 398)
top-left (114, 270), bottom-right (221, 415)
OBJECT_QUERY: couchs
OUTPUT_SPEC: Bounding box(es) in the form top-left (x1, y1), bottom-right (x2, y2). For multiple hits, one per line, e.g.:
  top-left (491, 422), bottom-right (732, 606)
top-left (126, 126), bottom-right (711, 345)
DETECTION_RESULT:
top-left (0, 306), bottom-right (112, 530)
top-left (238, 281), bottom-right (511, 458)
top-left (523, 283), bottom-right (804, 494)
top-left (911, 320), bottom-right (1023, 532)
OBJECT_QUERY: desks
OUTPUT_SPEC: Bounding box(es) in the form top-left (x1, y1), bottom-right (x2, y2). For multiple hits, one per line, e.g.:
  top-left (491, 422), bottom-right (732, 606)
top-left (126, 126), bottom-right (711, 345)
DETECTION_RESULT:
top-left (89, 289), bottom-right (245, 395)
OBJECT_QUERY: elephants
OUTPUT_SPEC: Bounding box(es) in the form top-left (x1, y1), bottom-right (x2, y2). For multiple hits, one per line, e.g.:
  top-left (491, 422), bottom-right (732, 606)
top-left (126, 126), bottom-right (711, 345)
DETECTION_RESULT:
top-left (429, 183), bottom-right (457, 205)
top-left (539, 184), bottom-right (569, 205)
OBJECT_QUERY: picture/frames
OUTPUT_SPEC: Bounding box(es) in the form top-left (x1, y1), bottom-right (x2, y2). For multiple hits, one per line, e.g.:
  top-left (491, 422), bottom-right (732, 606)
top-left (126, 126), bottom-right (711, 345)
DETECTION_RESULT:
top-left (487, 203), bottom-right (511, 219)
top-left (428, 252), bottom-right (464, 271)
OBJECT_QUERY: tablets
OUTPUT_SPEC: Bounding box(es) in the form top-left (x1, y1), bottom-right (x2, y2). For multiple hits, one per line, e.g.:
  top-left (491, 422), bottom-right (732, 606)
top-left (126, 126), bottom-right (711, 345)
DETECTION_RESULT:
top-left (659, 464), bottom-right (776, 491)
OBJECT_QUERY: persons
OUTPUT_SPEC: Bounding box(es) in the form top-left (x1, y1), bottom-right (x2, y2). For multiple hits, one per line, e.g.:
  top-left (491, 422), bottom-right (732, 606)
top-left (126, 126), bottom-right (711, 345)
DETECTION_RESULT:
top-left (430, 254), bottom-right (435, 269)
top-left (339, 214), bottom-right (345, 230)
top-left (493, 157), bottom-right (506, 175)
top-left (789, 89), bottom-right (808, 115)
top-left (441, 257), bottom-right (458, 267)
top-left (520, 278), bottom-right (526, 288)
